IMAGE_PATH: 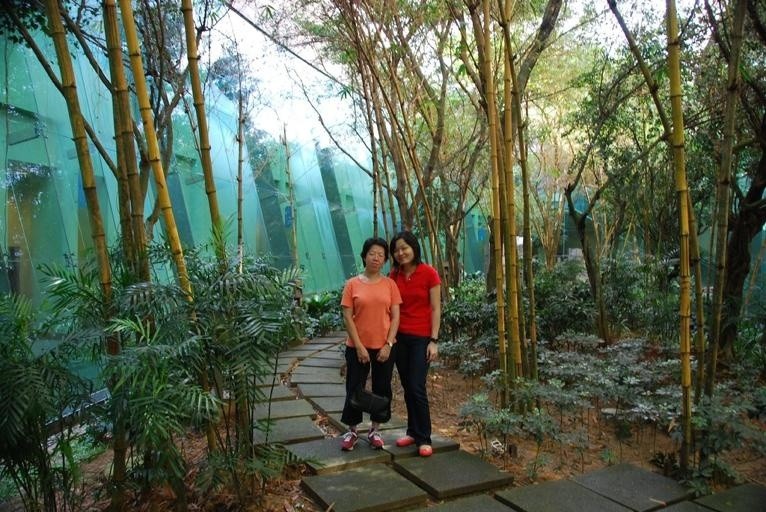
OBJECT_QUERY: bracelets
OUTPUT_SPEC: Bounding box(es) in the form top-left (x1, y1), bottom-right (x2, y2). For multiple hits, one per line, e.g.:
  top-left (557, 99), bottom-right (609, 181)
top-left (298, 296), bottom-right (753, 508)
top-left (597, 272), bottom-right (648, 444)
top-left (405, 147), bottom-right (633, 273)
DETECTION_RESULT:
top-left (386, 341), bottom-right (393, 347)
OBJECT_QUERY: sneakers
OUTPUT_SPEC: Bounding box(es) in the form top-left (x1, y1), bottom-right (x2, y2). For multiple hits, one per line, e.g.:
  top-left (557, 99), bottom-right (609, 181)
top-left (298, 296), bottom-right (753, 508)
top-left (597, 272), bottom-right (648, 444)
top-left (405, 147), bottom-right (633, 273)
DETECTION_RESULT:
top-left (367, 427), bottom-right (385, 449)
top-left (339, 429), bottom-right (360, 451)
top-left (396, 435), bottom-right (416, 447)
top-left (418, 444), bottom-right (433, 457)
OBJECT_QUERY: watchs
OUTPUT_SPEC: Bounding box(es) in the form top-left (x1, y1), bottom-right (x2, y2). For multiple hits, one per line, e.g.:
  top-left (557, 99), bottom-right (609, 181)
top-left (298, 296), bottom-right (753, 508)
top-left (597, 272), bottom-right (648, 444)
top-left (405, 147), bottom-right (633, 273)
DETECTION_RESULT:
top-left (430, 338), bottom-right (438, 343)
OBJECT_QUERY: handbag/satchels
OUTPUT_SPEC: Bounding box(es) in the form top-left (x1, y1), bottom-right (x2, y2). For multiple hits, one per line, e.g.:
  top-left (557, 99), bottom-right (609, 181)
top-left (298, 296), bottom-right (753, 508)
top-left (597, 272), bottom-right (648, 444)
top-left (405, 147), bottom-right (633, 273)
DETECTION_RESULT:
top-left (349, 362), bottom-right (390, 415)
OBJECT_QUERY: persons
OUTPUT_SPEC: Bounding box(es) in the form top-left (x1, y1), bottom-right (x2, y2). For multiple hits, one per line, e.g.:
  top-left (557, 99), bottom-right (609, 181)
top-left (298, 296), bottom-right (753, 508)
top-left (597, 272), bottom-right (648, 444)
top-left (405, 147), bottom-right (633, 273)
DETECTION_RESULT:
top-left (389, 231), bottom-right (442, 456)
top-left (340, 239), bottom-right (403, 450)
